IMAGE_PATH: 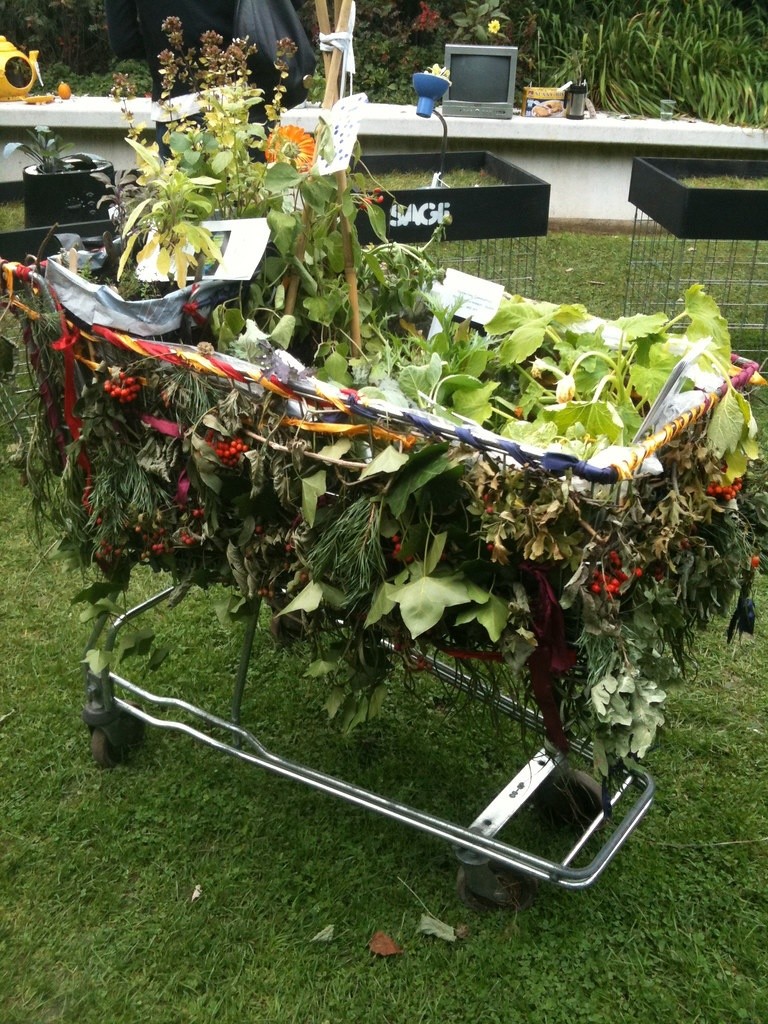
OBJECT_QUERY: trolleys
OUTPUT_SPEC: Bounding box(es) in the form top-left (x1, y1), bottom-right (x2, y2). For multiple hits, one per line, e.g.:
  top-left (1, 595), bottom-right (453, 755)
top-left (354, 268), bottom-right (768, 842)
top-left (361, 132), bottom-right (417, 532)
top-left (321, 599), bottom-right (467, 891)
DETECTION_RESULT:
top-left (0, 221), bottom-right (759, 918)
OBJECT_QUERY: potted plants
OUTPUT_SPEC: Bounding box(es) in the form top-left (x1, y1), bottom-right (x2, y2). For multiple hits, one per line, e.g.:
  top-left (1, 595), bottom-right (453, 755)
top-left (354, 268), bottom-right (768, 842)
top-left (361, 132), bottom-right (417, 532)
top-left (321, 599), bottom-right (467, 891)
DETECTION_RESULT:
top-left (0, 124), bottom-right (150, 292)
top-left (349, 150), bottom-right (550, 245)
top-left (629, 157), bottom-right (768, 240)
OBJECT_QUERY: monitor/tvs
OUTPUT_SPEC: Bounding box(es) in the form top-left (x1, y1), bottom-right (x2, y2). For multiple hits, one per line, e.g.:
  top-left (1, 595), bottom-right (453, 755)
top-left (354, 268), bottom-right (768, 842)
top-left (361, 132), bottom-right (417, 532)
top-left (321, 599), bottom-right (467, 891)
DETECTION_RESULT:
top-left (441, 44), bottom-right (519, 120)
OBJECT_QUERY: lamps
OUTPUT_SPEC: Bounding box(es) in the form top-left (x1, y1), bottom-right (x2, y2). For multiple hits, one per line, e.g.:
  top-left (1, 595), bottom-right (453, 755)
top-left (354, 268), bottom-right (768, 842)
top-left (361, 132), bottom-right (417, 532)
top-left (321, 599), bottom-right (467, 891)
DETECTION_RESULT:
top-left (412, 73), bottom-right (451, 187)
